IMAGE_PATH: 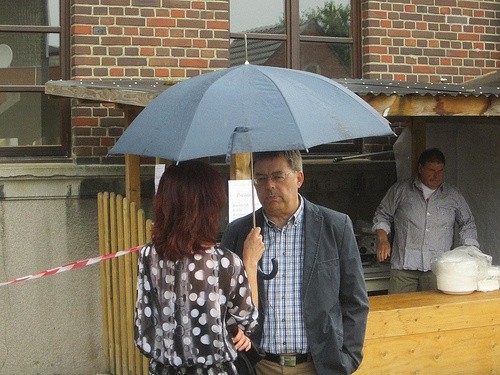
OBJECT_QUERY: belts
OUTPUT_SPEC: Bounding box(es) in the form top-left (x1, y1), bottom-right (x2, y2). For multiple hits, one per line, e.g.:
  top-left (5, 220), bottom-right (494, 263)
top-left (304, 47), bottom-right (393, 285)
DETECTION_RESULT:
top-left (265, 351), bottom-right (313, 366)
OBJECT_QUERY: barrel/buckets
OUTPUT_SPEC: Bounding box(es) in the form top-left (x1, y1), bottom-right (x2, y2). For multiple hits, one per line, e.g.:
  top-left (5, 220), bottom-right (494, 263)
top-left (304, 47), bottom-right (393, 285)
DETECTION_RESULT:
top-left (436, 257), bottom-right (479, 295)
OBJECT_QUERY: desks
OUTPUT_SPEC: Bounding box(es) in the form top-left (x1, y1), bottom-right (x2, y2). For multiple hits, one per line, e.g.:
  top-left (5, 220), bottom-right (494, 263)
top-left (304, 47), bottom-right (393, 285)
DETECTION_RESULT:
top-left (351, 282), bottom-right (500, 375)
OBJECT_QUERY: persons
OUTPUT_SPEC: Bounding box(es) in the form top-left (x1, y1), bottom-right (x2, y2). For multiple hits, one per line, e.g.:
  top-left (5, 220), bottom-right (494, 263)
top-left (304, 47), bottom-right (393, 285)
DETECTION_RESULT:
top-left (133, 161), bottom-right (267, 375)
top-left (372, 147), bottom-right (481, 294)
top-left (221, 151), bottom-right (370, 375)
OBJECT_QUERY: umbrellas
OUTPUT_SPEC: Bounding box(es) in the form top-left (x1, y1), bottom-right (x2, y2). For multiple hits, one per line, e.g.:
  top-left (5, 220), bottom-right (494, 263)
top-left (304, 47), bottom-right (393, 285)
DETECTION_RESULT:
top-left (108, 59), bottom-right (397, 227)
